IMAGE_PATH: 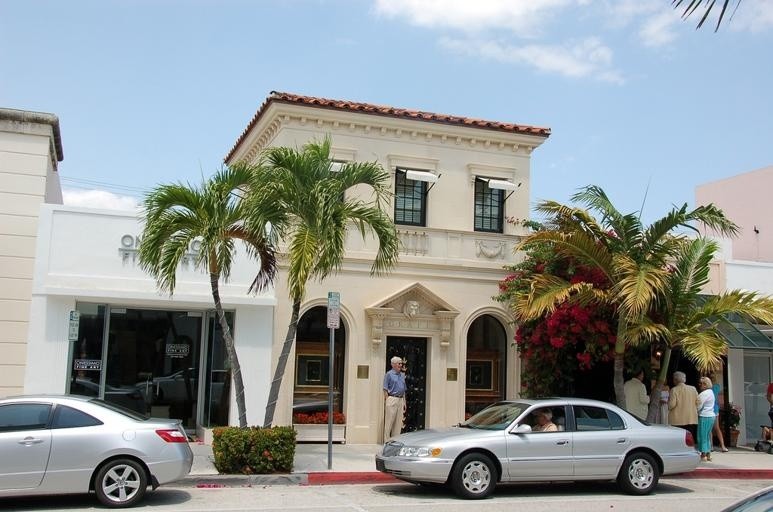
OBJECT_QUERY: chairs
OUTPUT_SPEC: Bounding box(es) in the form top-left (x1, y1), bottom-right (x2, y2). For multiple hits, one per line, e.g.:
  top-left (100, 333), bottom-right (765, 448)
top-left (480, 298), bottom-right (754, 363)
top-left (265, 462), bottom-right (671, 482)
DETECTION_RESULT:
top-left (553, 417), bottom-right (565, 432)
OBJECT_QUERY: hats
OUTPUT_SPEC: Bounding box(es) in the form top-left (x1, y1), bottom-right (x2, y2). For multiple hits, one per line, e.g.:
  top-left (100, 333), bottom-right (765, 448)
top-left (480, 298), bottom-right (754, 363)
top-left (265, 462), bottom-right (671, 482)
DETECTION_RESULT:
top-left (531, 408), bottom-right (552, 421)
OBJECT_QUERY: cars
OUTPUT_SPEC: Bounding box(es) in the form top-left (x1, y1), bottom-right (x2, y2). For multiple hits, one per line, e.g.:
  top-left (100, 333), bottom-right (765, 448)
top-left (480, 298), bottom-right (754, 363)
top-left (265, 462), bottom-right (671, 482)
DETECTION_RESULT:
top-left (0, 395), bottom-right (193, 507)
top-left (70, 368), bottom-right (228, 417)
top-left (376, 397), bottom-right (702, 499)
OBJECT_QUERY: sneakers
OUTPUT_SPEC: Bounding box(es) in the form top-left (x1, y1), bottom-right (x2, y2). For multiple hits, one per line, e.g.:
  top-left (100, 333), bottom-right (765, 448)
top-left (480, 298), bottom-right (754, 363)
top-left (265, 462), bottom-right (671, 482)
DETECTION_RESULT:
top-left (700, 447), bottom-right (729, 462)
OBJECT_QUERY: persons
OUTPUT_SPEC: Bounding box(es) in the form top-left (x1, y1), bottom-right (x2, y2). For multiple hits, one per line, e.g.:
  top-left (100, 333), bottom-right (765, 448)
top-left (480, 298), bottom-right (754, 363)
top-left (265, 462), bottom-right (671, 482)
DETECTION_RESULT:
top-left (706, 372), bottom-right (729, 452)
top-left (760, 426), bottom-right (773, 443)
top-left (622, 369), bottom-right (651, 421)
top-left (532, 408), bottom-right (557, 431)
top-left (668, 371), bottom-right (701, 444)
top-left (696, 376), bottom-right (717, 461)
top-left (766, 383), bottom-right (773, 427)
top-left (651, 375), bottom-right (669, 425)
top-left (656, 385), bottom-right (668, 402)
top-left (381, 355), bottom-right (407, 443)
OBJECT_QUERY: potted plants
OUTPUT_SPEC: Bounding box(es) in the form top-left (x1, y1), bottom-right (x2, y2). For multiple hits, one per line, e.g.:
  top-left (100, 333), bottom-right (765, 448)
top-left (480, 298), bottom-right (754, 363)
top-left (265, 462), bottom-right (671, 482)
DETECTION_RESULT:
top-left (720, 406), bottom-right (740, 447)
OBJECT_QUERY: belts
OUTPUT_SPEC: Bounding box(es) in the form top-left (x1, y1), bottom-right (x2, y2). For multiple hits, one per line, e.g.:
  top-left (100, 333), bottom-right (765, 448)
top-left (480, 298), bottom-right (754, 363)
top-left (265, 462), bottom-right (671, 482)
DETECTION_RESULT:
top-left (387, 394), bottom-right (404, 398)
